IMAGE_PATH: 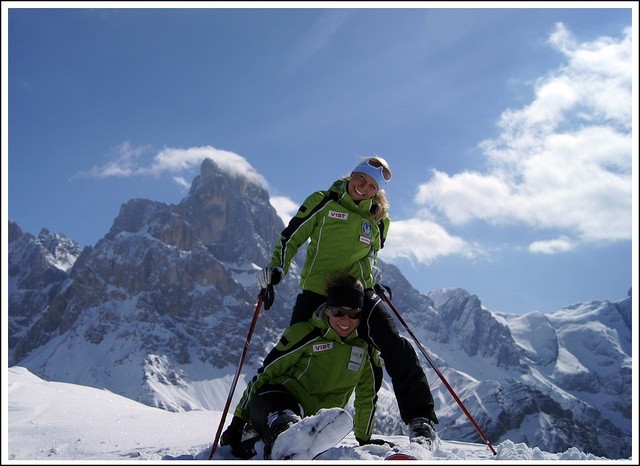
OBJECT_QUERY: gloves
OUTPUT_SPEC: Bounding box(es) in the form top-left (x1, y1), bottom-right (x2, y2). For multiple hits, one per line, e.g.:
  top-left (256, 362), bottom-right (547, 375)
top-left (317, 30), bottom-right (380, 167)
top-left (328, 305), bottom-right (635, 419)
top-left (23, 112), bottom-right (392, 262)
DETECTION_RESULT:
top-left (372, 266), bottom-right (382, 284)
top-left (359, 438), bottom-right (397, 450)
top-left (220, 417), bottom-right (246, 445)
top-left (257, 265), bottom-right (284, 288)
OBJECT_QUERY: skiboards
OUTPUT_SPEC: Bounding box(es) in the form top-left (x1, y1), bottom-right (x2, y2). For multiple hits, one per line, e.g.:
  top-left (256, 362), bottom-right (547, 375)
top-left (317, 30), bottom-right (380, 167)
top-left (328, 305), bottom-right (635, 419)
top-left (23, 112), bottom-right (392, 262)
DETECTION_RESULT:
top-left (384, 446), bottom-right (430, 461)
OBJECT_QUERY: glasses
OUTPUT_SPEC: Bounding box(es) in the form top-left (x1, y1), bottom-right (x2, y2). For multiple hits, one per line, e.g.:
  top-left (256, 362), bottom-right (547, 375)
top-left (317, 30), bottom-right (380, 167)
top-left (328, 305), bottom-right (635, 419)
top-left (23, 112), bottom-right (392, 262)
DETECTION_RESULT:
top-left (367, 157), bottom-right (392, 181)
top-left (331, 306), bottom-right (362, 320)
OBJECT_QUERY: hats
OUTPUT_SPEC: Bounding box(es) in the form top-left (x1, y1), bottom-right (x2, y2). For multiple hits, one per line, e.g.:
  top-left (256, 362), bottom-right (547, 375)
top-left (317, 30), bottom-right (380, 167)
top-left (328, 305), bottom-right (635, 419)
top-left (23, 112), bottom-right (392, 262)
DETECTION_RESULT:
top-left (352, 155), bottom-right (390, 192)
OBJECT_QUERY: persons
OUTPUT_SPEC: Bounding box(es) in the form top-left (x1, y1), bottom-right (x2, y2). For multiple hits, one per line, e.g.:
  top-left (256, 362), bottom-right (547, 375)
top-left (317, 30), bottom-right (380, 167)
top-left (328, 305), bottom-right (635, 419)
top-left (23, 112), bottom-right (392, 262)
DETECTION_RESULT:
top-left (256, 155), bottom-right (439, 452)
top-left (219, 274), bottom-right (400, 460)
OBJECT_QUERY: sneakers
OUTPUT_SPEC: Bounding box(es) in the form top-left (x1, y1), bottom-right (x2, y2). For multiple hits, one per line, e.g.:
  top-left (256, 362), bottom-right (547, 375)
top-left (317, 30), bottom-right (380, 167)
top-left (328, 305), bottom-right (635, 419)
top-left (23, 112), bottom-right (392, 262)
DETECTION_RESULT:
top-left (269, 407), bottom-right (354, 461)
top-left (409, 417), bottom-right (438, 451)
top-left (231, 436), bottom-right (264, 459)
